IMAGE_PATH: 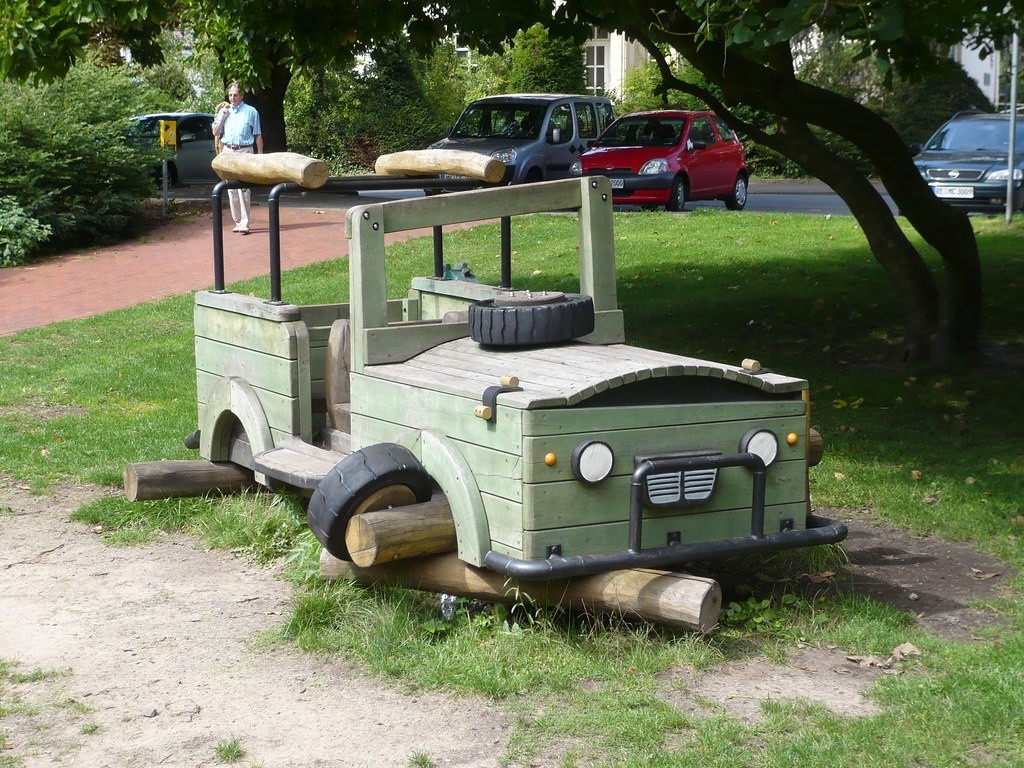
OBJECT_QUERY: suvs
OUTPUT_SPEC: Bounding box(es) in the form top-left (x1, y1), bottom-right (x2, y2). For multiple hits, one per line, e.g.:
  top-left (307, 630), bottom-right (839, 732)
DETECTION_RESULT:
top-left (909, 109), bottom-right (1023, 214)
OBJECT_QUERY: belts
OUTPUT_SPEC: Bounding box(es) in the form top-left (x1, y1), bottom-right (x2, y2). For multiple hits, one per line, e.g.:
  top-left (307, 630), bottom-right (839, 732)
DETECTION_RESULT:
top-left (224, 144), bottom-right (251, 150)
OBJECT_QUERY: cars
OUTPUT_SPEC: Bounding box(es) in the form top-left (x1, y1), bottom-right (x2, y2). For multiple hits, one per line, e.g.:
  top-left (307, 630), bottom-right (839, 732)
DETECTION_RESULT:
top-left (114, 112), bottom-right (225, 193)
top-left (567, 108), bottom-right (749, 212)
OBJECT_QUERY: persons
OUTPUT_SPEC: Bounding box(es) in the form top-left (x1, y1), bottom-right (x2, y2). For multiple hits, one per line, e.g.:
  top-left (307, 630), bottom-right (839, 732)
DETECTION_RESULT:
top-left (212, 83), bottom-right (263, 234)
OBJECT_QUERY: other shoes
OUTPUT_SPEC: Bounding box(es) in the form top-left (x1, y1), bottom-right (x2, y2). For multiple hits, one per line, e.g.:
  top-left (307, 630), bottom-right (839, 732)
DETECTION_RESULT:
top-left (239, 226), bottom-right (249, 234)
top-left (233, 225), bottom-right (240, 232)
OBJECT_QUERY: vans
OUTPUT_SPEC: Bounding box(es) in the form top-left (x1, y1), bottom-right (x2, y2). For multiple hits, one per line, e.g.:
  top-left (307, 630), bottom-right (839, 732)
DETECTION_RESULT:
top-left (427, 90), bottom-right (615, 197)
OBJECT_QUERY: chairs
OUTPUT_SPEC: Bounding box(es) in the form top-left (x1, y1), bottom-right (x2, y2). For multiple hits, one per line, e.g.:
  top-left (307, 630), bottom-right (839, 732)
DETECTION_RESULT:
top-left (654, 123), bottom-right (677, 144)
